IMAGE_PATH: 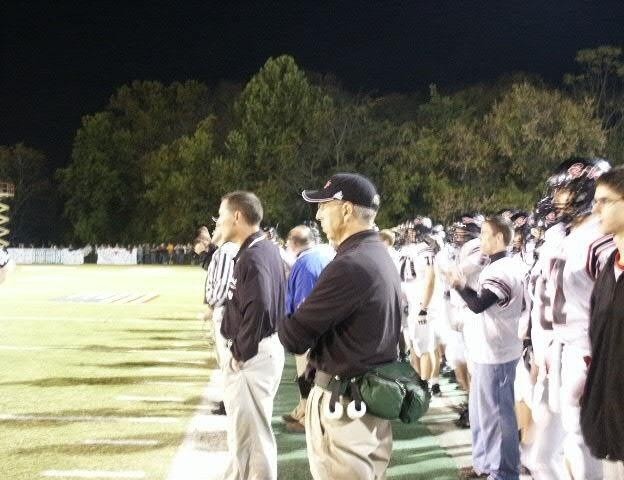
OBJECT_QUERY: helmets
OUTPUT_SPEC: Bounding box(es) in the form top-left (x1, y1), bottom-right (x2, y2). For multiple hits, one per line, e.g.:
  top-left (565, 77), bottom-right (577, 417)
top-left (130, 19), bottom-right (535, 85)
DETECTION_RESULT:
top-left (393, 159), bottom-right (613, 246)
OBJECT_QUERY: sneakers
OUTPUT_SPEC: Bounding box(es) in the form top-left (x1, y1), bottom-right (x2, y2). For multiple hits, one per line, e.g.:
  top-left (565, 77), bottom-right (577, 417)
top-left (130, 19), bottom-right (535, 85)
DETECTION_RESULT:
top-left (420, 354), bottom-right (471, 431)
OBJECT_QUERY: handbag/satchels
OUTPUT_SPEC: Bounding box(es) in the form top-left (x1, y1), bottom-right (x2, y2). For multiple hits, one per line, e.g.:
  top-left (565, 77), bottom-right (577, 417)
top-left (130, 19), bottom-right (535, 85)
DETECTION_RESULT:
top-left (357, 361), bottom-right (435, 425)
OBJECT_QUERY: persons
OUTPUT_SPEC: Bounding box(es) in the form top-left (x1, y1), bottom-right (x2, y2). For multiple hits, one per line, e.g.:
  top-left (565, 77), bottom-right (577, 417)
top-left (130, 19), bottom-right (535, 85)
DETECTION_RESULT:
top-left (0, 243), bottom-right (14, 284)
top-left (18, 241), bottom-right (203, 266)
top-left (199, 153), bottom-right (622, 480)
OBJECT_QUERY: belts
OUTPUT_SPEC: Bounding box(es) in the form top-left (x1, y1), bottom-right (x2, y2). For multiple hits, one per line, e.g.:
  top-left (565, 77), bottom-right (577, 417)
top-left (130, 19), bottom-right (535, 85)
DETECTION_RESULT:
top-left (315, 370), bottom-right (362, 402)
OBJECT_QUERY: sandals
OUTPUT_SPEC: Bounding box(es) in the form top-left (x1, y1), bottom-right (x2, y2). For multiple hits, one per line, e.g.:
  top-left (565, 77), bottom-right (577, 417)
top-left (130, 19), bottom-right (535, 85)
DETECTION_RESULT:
top-left (455, 462), bottom-right (491, 479)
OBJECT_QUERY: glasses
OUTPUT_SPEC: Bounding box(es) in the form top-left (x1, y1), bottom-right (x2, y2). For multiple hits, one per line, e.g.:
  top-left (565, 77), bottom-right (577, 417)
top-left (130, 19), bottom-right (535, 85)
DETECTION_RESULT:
top-left (590, 197), bottom-right (623, 206)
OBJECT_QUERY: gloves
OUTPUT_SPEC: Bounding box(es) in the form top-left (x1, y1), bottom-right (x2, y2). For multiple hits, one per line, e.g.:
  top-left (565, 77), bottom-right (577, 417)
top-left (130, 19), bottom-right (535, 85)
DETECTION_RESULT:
top-left (417, 312), bottom-right (428, 325)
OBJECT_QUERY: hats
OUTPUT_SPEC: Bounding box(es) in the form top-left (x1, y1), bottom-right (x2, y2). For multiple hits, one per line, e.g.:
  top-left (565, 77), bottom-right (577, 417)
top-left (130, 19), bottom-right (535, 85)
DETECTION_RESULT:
top-left (301, 171), bottom-right (381, 210)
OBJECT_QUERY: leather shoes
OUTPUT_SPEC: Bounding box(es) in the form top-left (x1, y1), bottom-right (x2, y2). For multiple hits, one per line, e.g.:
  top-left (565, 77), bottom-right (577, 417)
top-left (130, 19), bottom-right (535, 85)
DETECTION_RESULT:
top-left (282, 415), bottom-right (298, 423)
top-left (286, 421), bottom-right (304, 434)
